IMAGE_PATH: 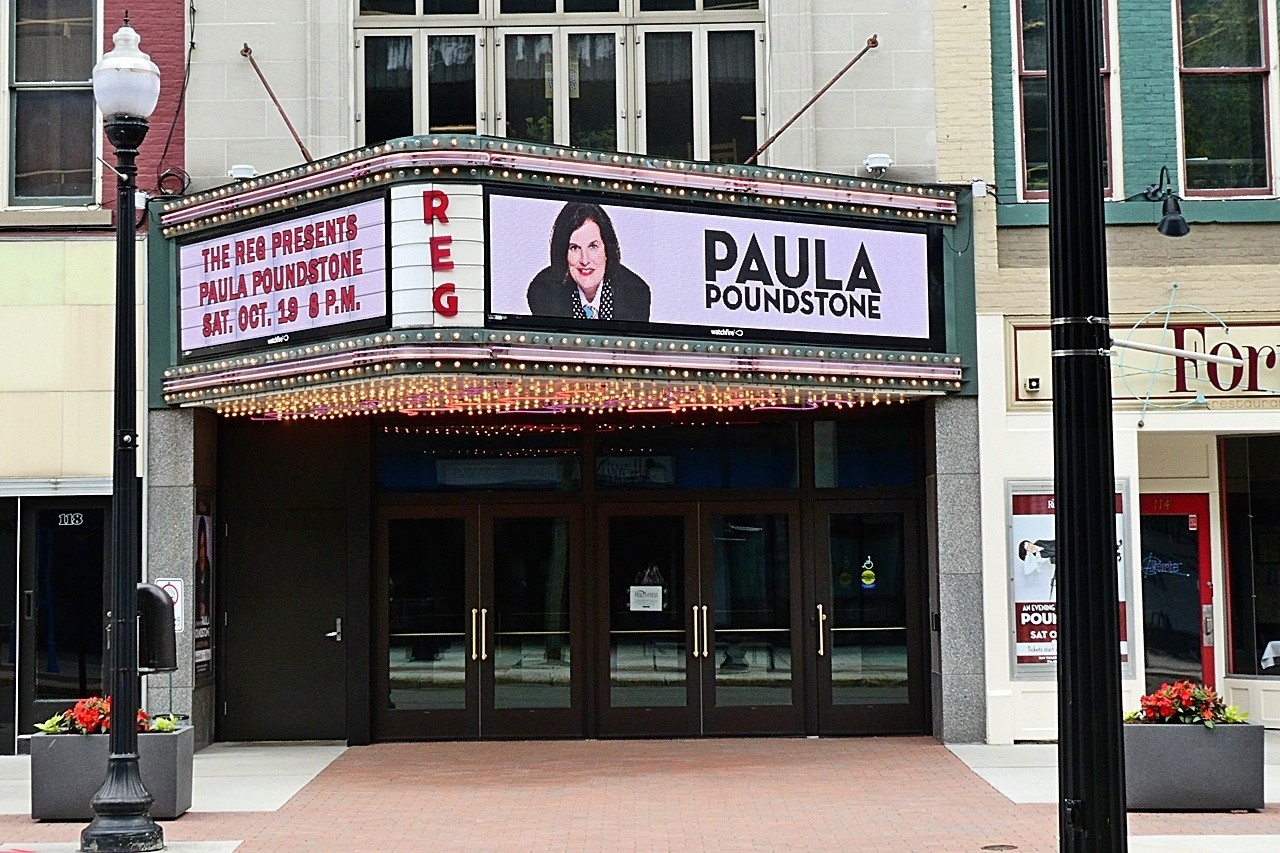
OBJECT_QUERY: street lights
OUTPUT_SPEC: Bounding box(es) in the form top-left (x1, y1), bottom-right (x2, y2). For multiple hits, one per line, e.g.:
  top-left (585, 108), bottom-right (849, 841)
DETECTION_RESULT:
top-left (80, 22), bottom-right (168, 853)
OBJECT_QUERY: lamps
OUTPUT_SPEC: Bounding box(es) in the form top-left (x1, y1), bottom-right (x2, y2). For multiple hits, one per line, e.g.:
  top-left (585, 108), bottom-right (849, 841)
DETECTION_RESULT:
top-left (1145, 165), bottom-right (1190, 237)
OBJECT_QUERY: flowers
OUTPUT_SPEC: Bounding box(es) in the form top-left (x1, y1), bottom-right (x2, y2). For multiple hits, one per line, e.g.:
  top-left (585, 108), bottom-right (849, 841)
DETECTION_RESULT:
top-left (33, 697), bottom-right (178, 735)
top-left (1124, 679), bottom-right (1252, 730)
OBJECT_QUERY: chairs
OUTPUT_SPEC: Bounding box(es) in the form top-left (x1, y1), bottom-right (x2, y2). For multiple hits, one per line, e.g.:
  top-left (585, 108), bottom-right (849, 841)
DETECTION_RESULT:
top-left (688, 138), bottom-right (738, 165)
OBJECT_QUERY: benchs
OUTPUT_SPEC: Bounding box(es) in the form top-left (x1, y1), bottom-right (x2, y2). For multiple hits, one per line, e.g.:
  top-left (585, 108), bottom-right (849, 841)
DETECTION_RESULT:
top-left (643, 609), bottom-right (777, 672)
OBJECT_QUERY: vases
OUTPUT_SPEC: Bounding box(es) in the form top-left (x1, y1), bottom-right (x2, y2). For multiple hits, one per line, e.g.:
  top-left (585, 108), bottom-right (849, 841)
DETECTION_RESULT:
top-left (31, 725), bottom-right (194, 821)
top-left (1123, 724), bottom-right (1265, 810)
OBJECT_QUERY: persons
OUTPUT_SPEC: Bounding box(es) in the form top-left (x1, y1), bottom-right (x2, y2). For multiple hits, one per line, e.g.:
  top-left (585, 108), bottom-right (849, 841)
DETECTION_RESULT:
top-left (527, 202), bottom-right (651, 320)
top-left (1018, 539), bottom-right (1056, 566)
